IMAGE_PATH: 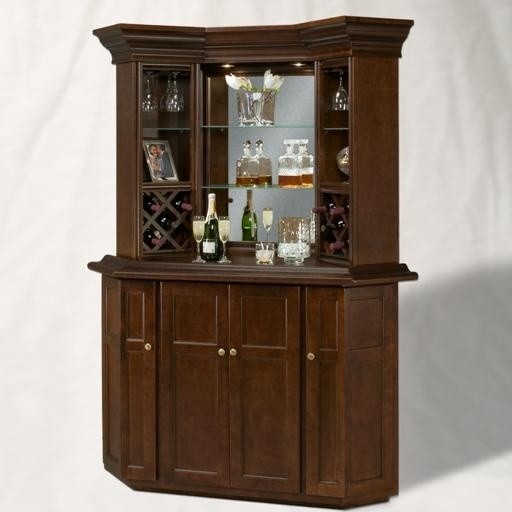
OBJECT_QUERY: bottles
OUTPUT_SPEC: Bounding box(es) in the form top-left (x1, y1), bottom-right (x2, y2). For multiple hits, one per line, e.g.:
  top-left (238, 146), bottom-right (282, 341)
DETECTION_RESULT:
top-left (143, 197), bottom-right (193, 247)
top-left (235, 135), bottom-right (314, 187)
top-left (313, 202), bottom-right (349, 252)
top-left (201, 193), bottom-right (222, 261)
top-left (242, 189), bottom-right (258, 241)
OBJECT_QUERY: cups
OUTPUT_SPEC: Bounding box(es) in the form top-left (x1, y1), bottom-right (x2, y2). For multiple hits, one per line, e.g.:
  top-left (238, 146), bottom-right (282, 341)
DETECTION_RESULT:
top-left (282, 243), bottom-right (305, 264)
top-left (277, 215), bottom-right (312, 259)
top-left (308, 206), bottom-right (316, 246)
top-left (254, 241), bottom-right (276, 266)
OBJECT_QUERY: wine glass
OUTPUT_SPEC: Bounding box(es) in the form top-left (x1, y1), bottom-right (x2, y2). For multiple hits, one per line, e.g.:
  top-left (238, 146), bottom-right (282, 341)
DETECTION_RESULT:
top-left (141, 70), bottom-right (185, 114)
top-left (191, 215), bottom-right (206, 263)
top-left (262, 207), bottom-right (274, 241)
top-left (331, 69), bottom-right (349, 111)
top-left (217, 216), bottom-right (233, 265)
